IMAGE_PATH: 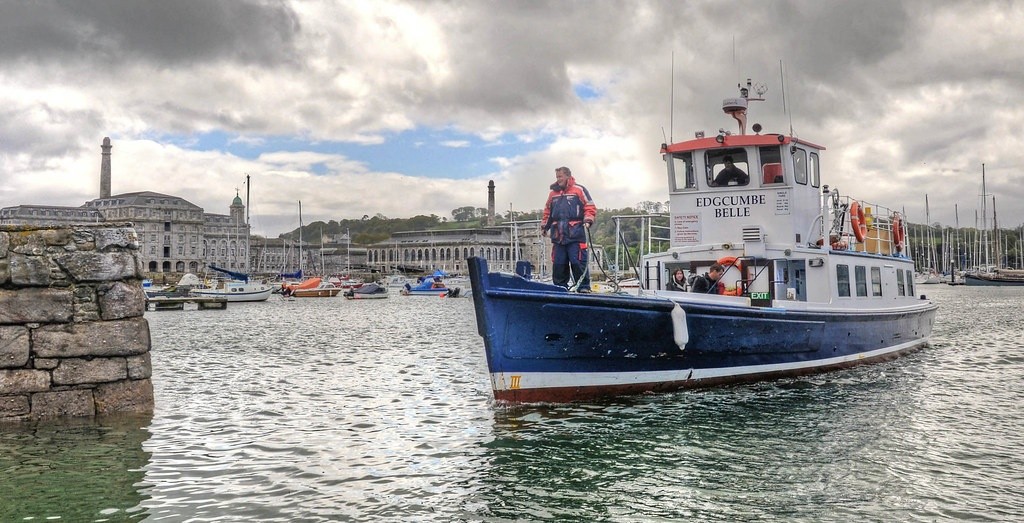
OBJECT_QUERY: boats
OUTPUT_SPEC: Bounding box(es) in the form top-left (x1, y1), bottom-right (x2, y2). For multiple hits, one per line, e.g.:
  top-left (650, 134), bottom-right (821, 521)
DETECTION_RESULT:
top-left (400, 276), bottom-right (450, 297)
top-left (606, 274), bottom-right (619, 282)
top-left (465, 77), bottom-right (937, 408)
top-left (343, 282), bottom-right (389, 299)
top-left (386, 275), bottom-right (421, 288)
top-left (143, 279), bottom-right (154, 289)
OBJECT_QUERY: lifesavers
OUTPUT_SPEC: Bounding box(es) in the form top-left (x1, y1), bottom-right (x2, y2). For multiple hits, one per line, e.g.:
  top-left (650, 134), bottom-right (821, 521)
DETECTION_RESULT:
top-left (893, 214), bottom-right (905, 250)
top-left (850, 201), bottom-right (867, 242)
top-left (716, 257), bottom-right (750, 295)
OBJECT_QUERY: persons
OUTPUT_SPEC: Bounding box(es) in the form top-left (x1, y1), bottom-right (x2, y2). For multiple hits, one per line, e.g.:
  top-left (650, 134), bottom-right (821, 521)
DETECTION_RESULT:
top-left (665, 264), bottom-right (725, 294)
top-left (540, 167), bottom-right (597, 293)
top-left (711, 156), bottom-right (748, 187)
top-left (444, 288), bottom-right (453, 298)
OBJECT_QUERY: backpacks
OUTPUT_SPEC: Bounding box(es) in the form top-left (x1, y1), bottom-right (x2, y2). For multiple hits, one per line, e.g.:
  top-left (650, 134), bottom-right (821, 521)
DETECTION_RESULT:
top-left (687, 274), bottom-right (709, 292)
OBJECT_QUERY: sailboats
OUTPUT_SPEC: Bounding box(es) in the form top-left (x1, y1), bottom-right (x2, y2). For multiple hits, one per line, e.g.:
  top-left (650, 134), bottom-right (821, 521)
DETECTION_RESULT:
top-left (188, 173), bottom-right (364, 302)
top-left (915, 163), bottom-right (1024, 285)
top-left (530, 215), bottom-right (552, 282)
top-left (618, 230), bottom-right (640, 288)
